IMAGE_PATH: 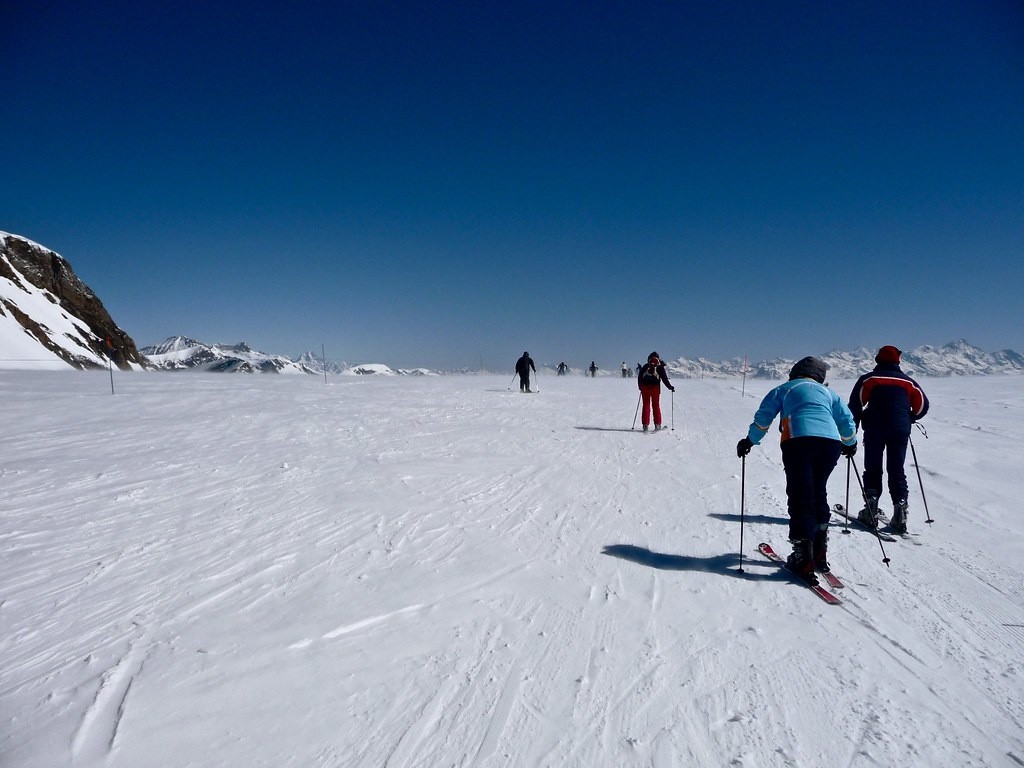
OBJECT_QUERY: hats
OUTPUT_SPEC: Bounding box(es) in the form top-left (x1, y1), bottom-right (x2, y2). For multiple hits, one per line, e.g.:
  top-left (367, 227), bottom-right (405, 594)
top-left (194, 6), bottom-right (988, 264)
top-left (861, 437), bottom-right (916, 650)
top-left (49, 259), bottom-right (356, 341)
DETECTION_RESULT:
top-left (876, 346), bottom-right (902, 366)
top-left (789, 357), bottom-right (827, 384)
top-left (647, 352), bottom-right (659, 362)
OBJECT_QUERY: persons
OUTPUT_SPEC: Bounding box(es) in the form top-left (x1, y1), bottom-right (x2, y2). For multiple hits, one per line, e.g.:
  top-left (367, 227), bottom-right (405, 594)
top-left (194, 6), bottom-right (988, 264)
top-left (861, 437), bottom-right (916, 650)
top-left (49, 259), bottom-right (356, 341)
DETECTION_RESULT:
top-left (737, 356), bottom-right (857, 584)
top-left (515, 351), bottom-right (536, 393)
top-left (589, 360), bottom-right (598, 377)
top-left (636, 350), bottom-right (674, 431)
top-left (621, 361), bottom-right (627, 377)
top-left (557, 362), bottom-right (567, 375)
top-left (848, 346), bottom-right (929, 534)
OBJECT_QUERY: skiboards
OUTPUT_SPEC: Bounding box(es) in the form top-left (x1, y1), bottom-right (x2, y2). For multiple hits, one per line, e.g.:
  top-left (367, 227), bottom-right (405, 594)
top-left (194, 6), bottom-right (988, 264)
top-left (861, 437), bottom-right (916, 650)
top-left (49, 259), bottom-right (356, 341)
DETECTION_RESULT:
top-left (759, 525), bottom-right (847, 608)
top-left (831, 501), bottom-right (924, 547)
top-left (642, 426), bottom-right (668, 436)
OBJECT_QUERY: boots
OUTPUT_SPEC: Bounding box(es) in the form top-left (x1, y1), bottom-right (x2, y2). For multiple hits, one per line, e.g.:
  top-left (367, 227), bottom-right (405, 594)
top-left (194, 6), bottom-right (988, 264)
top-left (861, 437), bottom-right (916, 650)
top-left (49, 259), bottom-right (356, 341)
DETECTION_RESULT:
top-left (860, 498), bottom-right (878, 528)
top-left (813, 530), bottom-right (828, 569)
top-left (890, 500), bottom-right (907, 534)
top-left (788, 539), bottom-right (816, 580)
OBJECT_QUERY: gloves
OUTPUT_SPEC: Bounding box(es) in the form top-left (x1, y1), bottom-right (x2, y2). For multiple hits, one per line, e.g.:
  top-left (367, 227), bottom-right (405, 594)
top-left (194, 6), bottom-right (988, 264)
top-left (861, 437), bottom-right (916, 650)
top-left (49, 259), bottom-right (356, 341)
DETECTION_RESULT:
top-left (737, 436), bottom-right (754, 457)
top-left (840, 441), bottom-right (858, 458)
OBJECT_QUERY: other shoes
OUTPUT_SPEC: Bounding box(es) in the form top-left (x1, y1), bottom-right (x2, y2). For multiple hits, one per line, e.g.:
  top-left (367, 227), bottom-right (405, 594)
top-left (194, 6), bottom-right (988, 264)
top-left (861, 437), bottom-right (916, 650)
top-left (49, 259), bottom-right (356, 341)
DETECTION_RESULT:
top-left (526, 388), bottom-right (531, 393)
top-left (520, 389), bottom-right (524, 393)
top-left (656, 425), bottom-right (661, 429)
top-left (643, 424), bottom-right (648, 431)
